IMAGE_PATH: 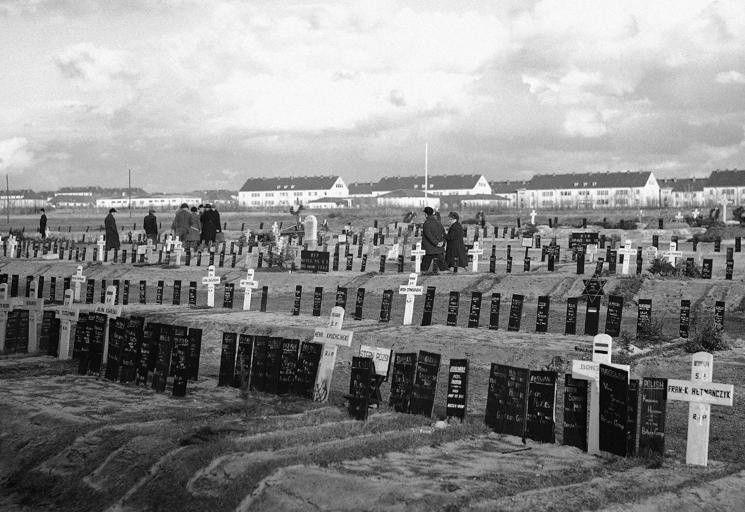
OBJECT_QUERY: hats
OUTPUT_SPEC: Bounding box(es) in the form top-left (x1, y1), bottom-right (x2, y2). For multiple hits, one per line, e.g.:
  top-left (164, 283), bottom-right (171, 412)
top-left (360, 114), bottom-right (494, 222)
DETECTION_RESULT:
top-left (149, 210), bottom-right (155, 214)
top-left (109, 208), bottom-right (117, 212)
top-left (423, 207), bottom-right (433, 214)
top-left (181, 204), bottom-right (216, 212)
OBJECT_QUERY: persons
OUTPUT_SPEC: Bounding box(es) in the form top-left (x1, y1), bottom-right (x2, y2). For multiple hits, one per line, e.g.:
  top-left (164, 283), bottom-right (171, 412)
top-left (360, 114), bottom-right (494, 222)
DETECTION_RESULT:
top-left (104, 207), bottom-right (122, 250)
top-left (143, 208), bottom-right (158, 248)
top-left (38, 208), bottom-right (47, 239)
top-left (171, 203), bottom-right (221, 245)
top-left (443, 210), bottom-right (471, 274)
top-left (419, 206), bottom-right (451, 272)
